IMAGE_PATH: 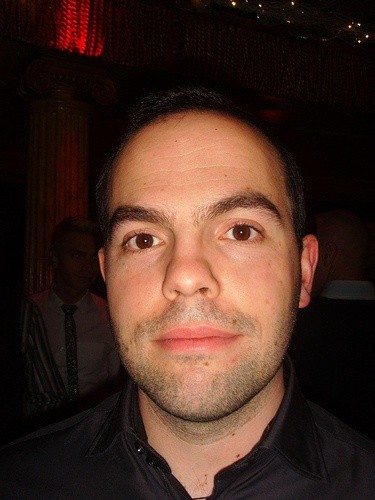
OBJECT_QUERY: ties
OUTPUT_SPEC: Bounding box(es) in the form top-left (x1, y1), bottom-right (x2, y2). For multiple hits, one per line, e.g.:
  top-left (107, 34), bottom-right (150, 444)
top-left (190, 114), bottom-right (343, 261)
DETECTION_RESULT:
top-left (59, 303), bottom-right (80, 407)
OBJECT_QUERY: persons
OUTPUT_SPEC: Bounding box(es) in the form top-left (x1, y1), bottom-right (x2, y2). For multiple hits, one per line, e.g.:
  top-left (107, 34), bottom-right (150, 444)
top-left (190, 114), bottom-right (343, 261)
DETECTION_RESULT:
top-left (0, 216), bottom-right (120, 430)
top-left (287, 209), bottom-right (375, 446)
top-left (0, 85), bottom-right (375, 500)
